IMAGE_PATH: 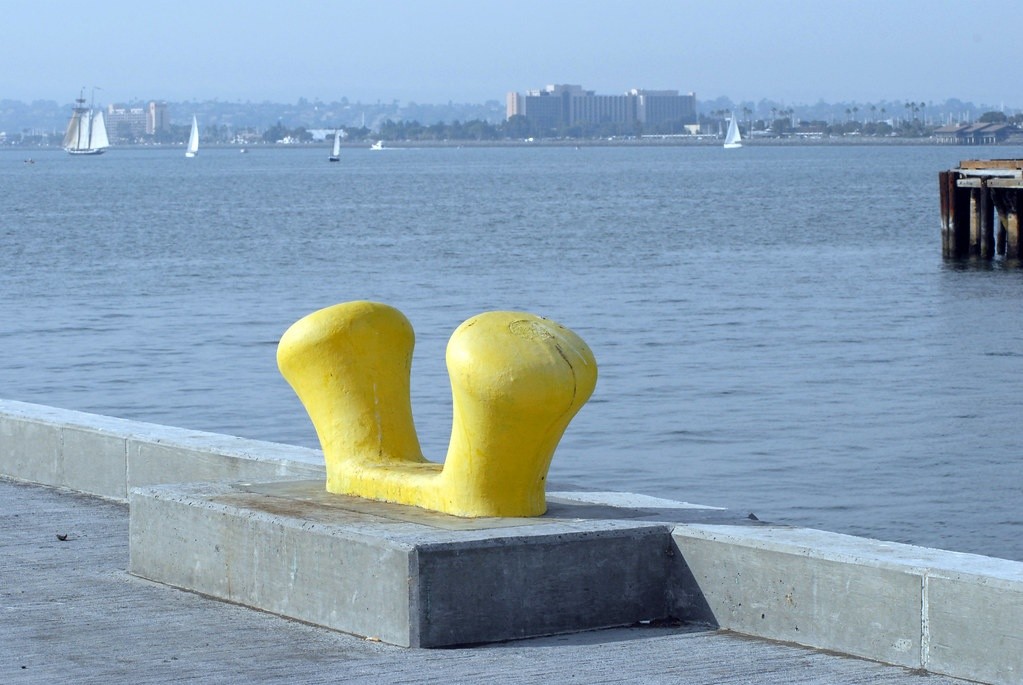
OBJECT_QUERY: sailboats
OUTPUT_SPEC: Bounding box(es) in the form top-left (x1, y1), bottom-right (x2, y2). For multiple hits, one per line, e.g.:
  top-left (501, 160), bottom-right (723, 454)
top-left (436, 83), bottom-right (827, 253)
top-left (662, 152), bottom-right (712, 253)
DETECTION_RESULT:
top-left (62, 105), bottom-right (110, 154)
top-left (723, 110), bottom-right (742, 149)
top-left (184, 114), bottom-right (199, 158)
top-left (328, 128), bottom-right (340, 162)
